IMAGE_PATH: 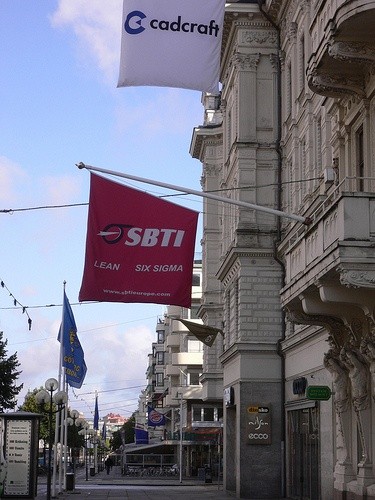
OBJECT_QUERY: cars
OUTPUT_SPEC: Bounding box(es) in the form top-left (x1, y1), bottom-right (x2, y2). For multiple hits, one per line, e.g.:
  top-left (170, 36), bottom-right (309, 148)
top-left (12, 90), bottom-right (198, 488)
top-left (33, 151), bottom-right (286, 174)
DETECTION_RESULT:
top-left (38, 458), bottom-right (49, 476)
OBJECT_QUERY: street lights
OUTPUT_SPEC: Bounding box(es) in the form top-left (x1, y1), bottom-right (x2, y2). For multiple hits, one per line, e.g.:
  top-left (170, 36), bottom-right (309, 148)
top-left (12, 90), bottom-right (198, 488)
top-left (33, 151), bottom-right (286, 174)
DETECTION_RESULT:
top-left (77, 423), bottom-right (95, 481)
top-left (34, 378), bottom-right (68, 500)
top-left (63, 409), bottom-right (84, 474)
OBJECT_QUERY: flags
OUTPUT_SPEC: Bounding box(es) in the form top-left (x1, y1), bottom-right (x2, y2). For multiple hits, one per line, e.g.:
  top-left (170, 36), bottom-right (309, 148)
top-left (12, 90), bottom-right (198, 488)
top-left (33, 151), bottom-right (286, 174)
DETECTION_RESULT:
top-left (78, 172), bottom-right (199, 310)
top-left (57, 292), bottom-right (88, 389)
top-left (147, 406), bottom-right (165, 426)
top-left (94, 398), bottom-right (100, 430)
top-left (116, 0), bottom-right (228, 94)
top-left (136, 429), bottom-right (148, 444)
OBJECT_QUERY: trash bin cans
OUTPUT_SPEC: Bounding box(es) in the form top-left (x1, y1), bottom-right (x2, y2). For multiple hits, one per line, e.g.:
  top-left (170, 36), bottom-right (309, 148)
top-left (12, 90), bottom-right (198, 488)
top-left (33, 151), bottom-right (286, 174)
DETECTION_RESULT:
top-left (205, 469), bottom-right (212, 483)
top-left (65, 473), bottom-right (75, 491)
top-left (90, 467), bottom-right (95, 477)
